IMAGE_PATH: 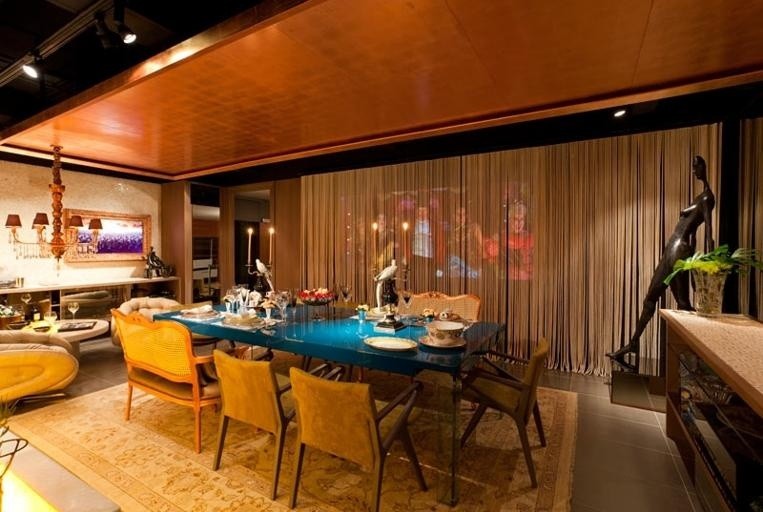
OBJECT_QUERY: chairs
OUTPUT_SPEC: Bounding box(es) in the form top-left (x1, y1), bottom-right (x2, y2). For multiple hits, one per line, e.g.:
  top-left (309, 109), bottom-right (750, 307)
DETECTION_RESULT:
top-left (0, 330), bottom-right (80, 414)
top-left (398, 288), bottom-right (480, 323)
top-left (288, 364), bottom-right (427, 512)
top-left (109, 296), bottom-right (181, 346)
top-left (110, 307), bottom-right (250, 454)
top-left (459, 339), bottom-right (549, 488)
top-left (212, 349), bottom-right (333, 501)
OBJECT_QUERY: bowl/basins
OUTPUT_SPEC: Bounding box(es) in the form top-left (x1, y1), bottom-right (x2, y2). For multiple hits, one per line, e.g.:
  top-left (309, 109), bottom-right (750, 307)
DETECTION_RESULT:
top-left (8, 320), bottom-right (27, 330)
top-left (424, 320), bottom-right (466, 344)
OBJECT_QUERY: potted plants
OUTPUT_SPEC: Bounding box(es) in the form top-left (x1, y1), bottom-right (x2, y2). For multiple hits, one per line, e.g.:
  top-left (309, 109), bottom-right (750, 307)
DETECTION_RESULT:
top-left (663, 244), bottom-right (763, 319)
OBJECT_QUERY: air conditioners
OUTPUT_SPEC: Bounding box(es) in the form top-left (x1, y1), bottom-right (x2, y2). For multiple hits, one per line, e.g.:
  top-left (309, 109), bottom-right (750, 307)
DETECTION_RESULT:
top-left (192, 204), bottom-right (220, 221)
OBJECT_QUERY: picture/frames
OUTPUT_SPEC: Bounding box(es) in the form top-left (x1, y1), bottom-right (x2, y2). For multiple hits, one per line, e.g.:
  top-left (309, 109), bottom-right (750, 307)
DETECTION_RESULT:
top-left (62, 209), bottom-right (152, 263)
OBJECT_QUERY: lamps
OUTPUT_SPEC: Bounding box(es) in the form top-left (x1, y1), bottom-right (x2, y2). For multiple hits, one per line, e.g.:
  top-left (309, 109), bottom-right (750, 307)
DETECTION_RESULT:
top-left (23, 50), bottom-right (42, 79)
top-left (118, 23), bottom-right (137, 45)
top-left (95, 17), bottom-right (121, 50)
top-left (5, 146), bottom-right (103, 260)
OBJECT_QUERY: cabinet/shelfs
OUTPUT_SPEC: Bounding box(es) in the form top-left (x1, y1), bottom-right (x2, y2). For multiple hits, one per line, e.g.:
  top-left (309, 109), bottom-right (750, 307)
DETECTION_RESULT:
top-left (0, 273), bottom-right (182, 321)
top-left (659, 309), bottom-right (763, 512)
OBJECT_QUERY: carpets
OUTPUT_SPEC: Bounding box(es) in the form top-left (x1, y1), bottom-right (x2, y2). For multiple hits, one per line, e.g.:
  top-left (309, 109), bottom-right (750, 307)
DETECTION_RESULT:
top-left (0, 369), bottom-right (579, 512)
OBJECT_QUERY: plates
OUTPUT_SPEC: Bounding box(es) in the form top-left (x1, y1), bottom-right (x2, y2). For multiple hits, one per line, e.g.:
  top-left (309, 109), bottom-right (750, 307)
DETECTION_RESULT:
top-left (220, 317), bottom-right (266, 328)
top-left (363, 336), bottom-right (417, 352)
top-left (418, 335), bottom-right (467, 349)
top-left (182, 310), bottom-right (218, 318)
top-left (34, 325), bottom-right (50, 331)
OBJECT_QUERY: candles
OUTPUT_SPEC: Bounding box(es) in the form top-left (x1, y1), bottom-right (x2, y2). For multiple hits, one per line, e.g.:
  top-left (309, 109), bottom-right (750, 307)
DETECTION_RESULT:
top-left (269, 227), bottom-right (274, 264)
top-left (402, 223), bottom-right (409, 265)
top-left (247, 228), bottom-right (253, 265)
top-left (372, 222), bottom-right (379, 268)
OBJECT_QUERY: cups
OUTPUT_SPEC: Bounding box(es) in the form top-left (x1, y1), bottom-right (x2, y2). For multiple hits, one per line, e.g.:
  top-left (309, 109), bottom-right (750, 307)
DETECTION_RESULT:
top-left (43, 312), bottom-right (58, 326)
top-left (20, 294), bottom-right (32, 304)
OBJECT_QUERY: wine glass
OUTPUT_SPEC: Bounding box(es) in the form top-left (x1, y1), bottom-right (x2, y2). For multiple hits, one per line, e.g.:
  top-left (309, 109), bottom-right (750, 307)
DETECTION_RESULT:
top-left (290, 288), bottom-right (300, 327)
top-left (274, 290), bottom-right (290, 327)
top-left (332, 283), bottom-right (351, 315)
top-left (67, 301), bottom-right (80, 323)
top-left (297, 291), bottom-right (334, 321)
top-left (225, 282), bottom-right (249, 312)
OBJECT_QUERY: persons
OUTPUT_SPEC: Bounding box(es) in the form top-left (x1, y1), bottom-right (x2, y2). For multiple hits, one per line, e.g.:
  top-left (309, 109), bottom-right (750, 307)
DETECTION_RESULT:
top-left (605, 154), bottom-right (714, 362)
top-left (345, 193), bottom-right (533, 279)
top-left (77, 231), bottom-right (143, 254)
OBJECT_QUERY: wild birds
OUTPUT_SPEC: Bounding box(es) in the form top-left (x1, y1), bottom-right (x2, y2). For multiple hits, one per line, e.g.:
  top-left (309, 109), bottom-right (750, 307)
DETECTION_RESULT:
top-left (255, 258), bottom-right (277, 295)
top-left (373, 259), bottom-right (399, 313)
